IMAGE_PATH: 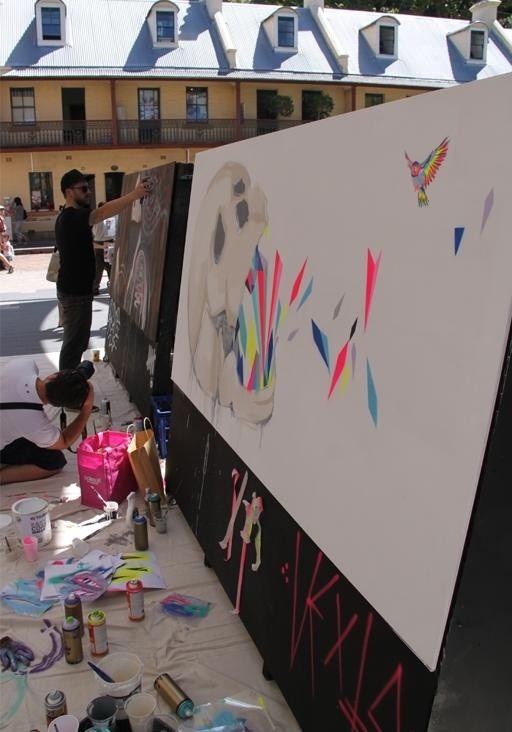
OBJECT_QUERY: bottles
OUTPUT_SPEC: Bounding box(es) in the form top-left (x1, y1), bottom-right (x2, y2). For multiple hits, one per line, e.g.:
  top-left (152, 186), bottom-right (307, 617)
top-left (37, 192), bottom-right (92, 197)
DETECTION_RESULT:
top-left (44, 689), bottom-right (67, 724)
top-left (134, 414), bottom-right (144, 432)
top-left (144, 487), bottom-right (162, 526)
top-left (133, 515), bottom-right (150, 550)
top-left (101, 396), bottom-right (111, 416)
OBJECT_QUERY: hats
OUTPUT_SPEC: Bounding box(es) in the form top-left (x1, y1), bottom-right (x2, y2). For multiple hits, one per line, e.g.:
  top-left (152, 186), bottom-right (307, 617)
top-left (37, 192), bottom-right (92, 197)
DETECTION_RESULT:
top-left (60, 168), bottom-right (95, 191)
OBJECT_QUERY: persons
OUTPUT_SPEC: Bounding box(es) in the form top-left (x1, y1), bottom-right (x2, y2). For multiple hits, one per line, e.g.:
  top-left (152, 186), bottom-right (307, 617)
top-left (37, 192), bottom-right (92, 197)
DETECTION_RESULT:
top-left (93, 199), bottom-right (113, 297)
top-left (0, 357), bottom-right (95, 489)
top-left (10, 195), bottom-right (27, 220)
top-left (1, 230), bottom-right (15, 269)
top-left (0, 253), bottom-right (14, 274)
top-left (0, 204), bottom-right (15, 270)
top-left (53, 167), bottom-right (154, 417)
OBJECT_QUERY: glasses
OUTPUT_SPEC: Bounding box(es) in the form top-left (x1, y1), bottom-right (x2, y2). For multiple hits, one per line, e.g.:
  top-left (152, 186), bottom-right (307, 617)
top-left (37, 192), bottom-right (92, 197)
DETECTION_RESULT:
top-left (68, 185), bottom-right (93, 193)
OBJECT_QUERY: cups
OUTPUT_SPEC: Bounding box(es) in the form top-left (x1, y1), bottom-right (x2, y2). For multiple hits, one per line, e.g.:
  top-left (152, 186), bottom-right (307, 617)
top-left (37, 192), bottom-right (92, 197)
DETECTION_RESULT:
top-left (155, 517), bottom-right (168, 533)
top-left (21, 536), bottom-right (38, 561)
top-left (79, 652), bottom-right (155, 732)
top-left (70, 538), bottom-right (90, 553)
top-left (92, 349), bottom-right (100, 361)
top-left (47, 714), bottom-right (79, 732)
top-left (103, 502), bottom-right (119, 521)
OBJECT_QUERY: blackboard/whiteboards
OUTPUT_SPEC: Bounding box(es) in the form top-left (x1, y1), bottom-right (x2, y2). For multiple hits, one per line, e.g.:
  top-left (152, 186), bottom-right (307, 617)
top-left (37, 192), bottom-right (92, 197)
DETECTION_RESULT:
top-left (29, 172), bottom-right (54, 210)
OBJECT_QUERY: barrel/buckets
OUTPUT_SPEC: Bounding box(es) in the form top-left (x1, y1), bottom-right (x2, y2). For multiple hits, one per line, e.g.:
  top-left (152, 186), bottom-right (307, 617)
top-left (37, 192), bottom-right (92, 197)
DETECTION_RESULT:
top-left (92, 653), bottom-right (145, 723)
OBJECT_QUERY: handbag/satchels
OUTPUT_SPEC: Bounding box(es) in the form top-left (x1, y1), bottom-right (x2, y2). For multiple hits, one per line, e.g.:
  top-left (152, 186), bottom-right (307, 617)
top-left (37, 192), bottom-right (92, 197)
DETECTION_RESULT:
top-left (46, 251), bottom-right (61, 282)
top-left (77, 417), bottom-right (164, 510)
